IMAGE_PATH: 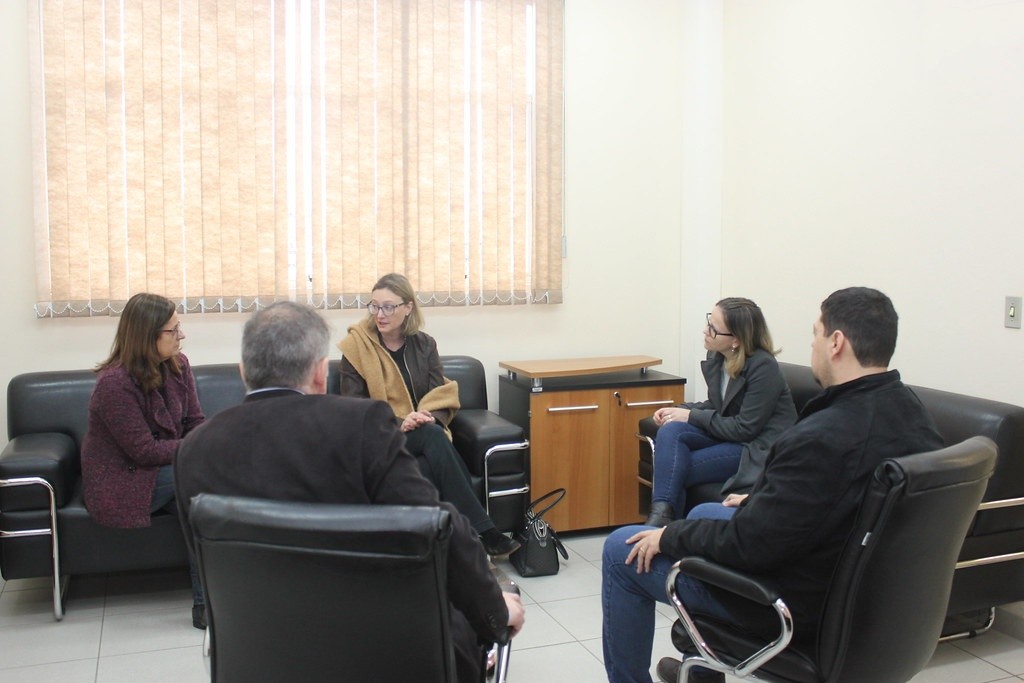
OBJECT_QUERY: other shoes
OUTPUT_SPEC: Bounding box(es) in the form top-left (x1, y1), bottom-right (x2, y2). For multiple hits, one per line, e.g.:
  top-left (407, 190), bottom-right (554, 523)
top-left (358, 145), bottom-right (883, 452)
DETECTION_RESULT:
top-left (485, 534), bottom-right (522, 557)
top-left (656, 657), bottom-right (725, 683)
top-left (192, 603), bottom-right (208, 630)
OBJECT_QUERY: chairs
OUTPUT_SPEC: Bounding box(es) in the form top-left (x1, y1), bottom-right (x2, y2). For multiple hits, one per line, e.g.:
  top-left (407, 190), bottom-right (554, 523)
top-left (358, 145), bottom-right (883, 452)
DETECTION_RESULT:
top-left (190, 493), bottom-right (520, 683)
top-left (666, 435), bottom-right (998, 683)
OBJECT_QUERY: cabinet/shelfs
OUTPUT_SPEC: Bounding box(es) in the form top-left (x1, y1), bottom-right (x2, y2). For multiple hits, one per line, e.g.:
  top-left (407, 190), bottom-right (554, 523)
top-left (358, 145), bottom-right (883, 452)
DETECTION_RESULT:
top-left (498, 355), bottom-right (687, 532)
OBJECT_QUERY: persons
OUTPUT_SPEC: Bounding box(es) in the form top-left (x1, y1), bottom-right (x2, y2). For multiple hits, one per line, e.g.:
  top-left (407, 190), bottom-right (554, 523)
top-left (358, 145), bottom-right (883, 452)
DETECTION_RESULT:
top-left (338, 273), bottom-right (523, 560)
top-left (600, 286), bottom-right (952, 683)
top-left (173, 298), bottom-right (527, 683)
top-left (644, 296), bottom-right (800, 528)
top-left (80, 292), bottom-right (210, 633)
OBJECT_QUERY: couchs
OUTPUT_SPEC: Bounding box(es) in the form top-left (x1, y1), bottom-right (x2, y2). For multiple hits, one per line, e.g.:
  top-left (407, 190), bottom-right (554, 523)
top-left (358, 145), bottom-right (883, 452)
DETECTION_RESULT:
top-left (634, 363), bottom-right (1024, 642)
top-left (0, 354), bottom-right (527, 622)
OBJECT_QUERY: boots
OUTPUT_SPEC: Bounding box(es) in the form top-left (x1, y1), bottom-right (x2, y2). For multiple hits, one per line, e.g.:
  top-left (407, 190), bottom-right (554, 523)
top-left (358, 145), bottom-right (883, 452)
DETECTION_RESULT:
top-left (643, 500), bottom-right (674, 528)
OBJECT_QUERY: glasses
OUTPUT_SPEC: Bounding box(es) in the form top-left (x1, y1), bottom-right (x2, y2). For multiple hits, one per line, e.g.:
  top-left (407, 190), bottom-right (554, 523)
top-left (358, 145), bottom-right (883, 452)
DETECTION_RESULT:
top-left (706, 311), bottom-right (734, 339)
top-left (156, 321), bottom-right (181, 335)
top-left (366, 300), bottom-right (408, 316)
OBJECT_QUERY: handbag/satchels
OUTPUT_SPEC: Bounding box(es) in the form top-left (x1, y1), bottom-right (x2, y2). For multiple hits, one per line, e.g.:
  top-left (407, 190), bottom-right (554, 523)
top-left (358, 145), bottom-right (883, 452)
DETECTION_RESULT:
top-left (509, 488), bottom-right (568, 577)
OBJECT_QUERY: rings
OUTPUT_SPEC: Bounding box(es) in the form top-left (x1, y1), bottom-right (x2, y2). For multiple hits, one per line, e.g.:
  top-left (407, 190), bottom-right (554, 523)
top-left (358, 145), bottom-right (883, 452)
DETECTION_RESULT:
top-left (640, 548), bottom-right (645, 555)
top-left (669, 419), bottom-right (672, 422)
top-left (668, 415), bottom-right (672, 419)
